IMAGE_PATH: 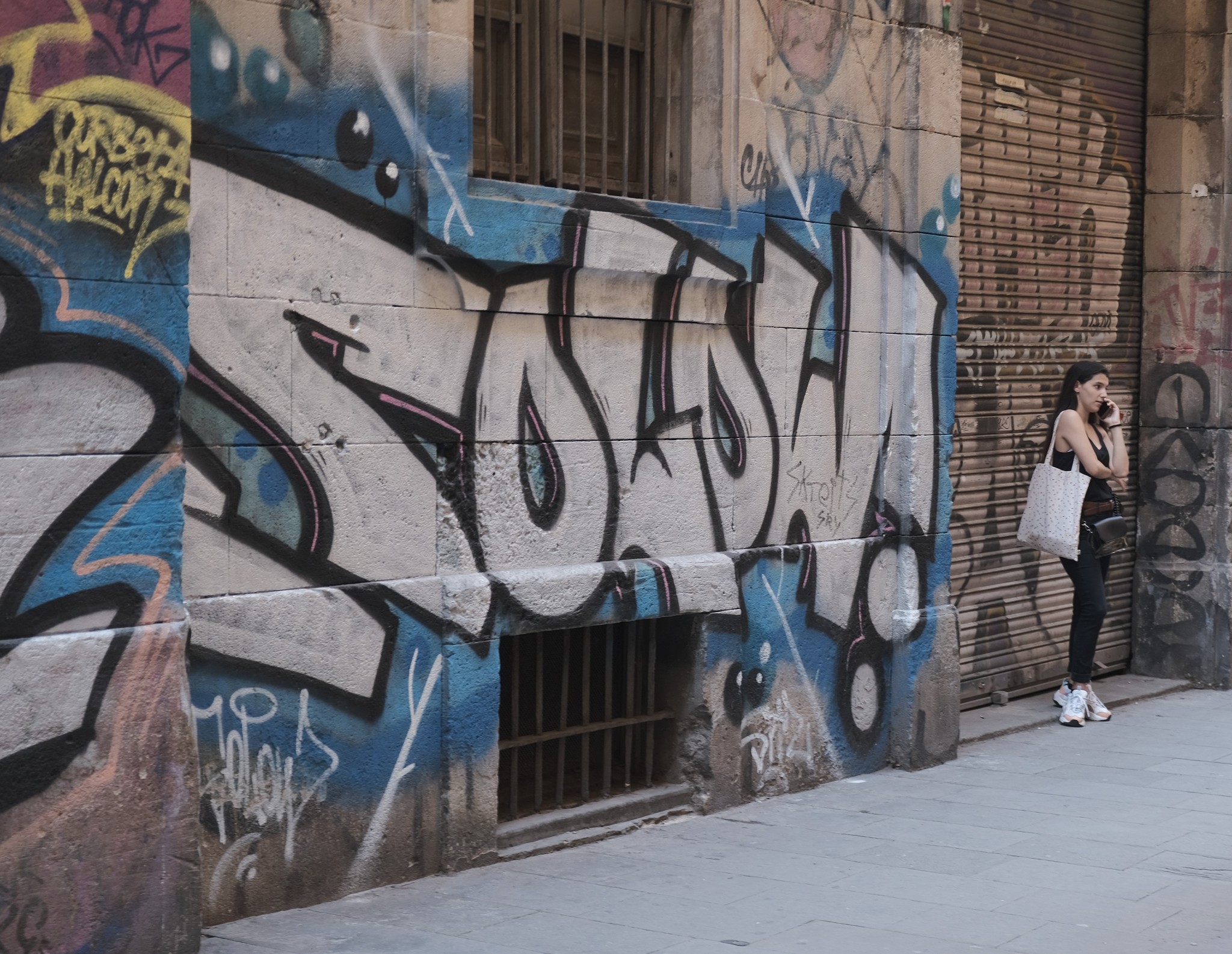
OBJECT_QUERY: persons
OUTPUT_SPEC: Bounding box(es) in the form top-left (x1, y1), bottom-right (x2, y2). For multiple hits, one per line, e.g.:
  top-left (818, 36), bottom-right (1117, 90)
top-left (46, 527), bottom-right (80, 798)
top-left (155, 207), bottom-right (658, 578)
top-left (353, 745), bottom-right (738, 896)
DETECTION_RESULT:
top-left (1045, 360), bottom-right (1128, 727)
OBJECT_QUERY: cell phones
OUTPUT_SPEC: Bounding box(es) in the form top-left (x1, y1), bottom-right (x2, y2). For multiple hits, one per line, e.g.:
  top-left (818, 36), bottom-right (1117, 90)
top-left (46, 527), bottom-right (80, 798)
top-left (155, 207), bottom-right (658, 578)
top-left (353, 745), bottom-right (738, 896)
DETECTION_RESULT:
top-left (1097, 401), bottom-right (1109, 419)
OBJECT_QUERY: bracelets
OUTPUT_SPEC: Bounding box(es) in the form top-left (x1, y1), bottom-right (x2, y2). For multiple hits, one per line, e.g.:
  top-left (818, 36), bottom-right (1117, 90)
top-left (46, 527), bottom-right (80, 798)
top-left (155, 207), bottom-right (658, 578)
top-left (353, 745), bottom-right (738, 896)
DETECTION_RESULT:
top-left (1108, 423), bottom-right (1121, 430)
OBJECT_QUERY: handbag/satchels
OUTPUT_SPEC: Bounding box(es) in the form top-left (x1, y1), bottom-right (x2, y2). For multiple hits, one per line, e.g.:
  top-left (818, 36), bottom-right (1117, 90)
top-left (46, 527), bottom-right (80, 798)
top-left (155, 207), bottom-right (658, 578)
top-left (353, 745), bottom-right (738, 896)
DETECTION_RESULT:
top-left (1016, 411), bottom-right (1092, 562)
top-left (1089, 514), bottom-right (1128, 559)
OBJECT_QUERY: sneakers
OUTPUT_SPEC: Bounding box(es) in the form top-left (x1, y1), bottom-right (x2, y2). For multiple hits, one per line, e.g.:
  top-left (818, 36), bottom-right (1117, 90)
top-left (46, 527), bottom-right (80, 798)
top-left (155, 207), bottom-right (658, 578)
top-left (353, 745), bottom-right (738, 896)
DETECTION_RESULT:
top-left (1052, 678), bottom-right (1112, 722)
top-left (1059, 685), bottom-right (1090, 727)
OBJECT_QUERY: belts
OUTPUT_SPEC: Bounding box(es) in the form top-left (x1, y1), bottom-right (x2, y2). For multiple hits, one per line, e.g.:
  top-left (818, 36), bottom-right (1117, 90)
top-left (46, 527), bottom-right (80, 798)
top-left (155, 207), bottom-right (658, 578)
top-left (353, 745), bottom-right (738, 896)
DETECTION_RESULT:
top-left (1082, 498), bottom-right (1121, 516)
